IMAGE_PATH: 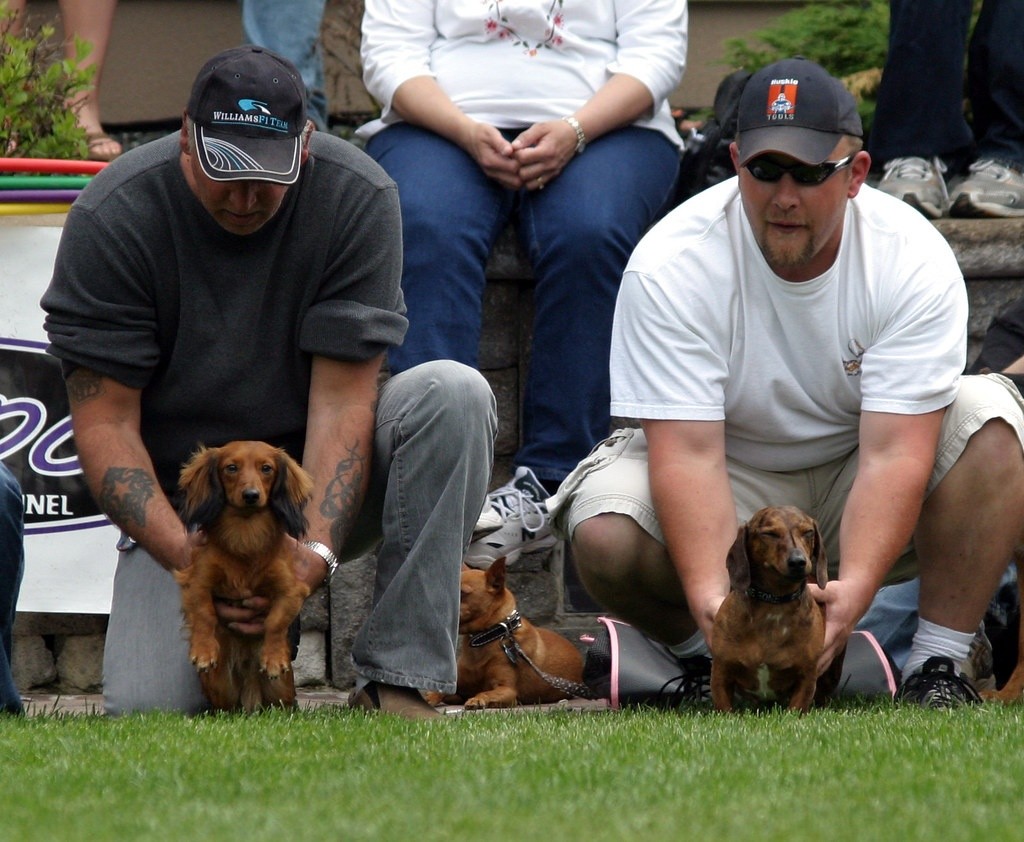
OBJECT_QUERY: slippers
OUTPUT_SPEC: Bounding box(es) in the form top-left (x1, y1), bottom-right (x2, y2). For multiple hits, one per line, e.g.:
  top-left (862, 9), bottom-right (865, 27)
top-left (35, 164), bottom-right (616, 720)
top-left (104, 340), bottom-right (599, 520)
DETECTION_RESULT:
top-left (83, 132), bottom-right (122, 160)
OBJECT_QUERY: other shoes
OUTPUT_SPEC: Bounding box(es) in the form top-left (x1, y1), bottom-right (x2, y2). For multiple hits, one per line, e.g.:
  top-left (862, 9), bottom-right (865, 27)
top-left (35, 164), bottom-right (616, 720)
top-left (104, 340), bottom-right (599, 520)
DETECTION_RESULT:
top-left (349, 681), bottom-right (440, 720)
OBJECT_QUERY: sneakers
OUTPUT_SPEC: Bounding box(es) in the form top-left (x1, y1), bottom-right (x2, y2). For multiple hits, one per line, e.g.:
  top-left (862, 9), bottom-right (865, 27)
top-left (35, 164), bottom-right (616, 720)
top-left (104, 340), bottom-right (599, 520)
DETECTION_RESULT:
top-left (878, 155), bottom-right (950, 217)
top-left (470, 493), bottom-right (504, 543)
top-left (893, 655), bottom-right (983, 711)
top-left (657, 655), bottom-right (763, 714)
top-left (948, 159), bottom-right (1024, 217)
top-left (464, 466), bottom-right (558, 571)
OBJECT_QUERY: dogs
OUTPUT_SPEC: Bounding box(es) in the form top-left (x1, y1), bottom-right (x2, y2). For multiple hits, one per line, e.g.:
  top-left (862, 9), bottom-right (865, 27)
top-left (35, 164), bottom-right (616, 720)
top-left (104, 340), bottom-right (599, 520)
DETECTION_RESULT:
top-left (709, 503), bottom-right (828, 714)
top-left (425, 553), bottom-right (585, 710)
top-left (174, 439), bottom-right (316, 713)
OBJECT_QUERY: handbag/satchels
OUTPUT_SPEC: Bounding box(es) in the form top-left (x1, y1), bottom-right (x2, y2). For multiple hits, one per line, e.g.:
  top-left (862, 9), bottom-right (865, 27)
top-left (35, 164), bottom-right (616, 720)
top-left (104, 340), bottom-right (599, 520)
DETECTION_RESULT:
top-left (672, 65), bottom-right (755, 204)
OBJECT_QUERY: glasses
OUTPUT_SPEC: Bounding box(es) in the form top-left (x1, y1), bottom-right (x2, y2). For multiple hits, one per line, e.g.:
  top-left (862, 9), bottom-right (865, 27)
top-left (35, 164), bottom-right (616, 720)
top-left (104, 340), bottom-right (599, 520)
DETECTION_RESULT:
top-left (745, 155), bottom-right (852, 186)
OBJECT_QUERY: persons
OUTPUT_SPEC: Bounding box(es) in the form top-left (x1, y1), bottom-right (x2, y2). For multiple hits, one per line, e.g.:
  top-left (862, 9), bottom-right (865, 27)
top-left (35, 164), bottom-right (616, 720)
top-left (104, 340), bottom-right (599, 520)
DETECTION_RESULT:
top-left (871, 0), bottom-right (1024, 216)
top-left (354, 0), bottom-right (689, 569)
top-left (848, 293), bottom-right (1024, 702)
top-left (0, 460), bottom-right (27, 722)
top-left (61, 46), bottom-right (499, 718)
top-left (241, 0), bottom-right (328, 133)
top-left (0, 0), bottom-right (123, 160)
top-left (546, 54), bottom-right (1024, 705)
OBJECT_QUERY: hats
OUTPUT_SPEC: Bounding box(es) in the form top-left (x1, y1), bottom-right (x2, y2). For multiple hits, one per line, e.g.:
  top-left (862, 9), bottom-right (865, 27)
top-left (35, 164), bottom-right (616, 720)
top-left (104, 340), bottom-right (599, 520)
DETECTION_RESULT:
top-left (186, 45), bottom-right (308, 184)
top-left (736, 55), bottom-right (865, 167)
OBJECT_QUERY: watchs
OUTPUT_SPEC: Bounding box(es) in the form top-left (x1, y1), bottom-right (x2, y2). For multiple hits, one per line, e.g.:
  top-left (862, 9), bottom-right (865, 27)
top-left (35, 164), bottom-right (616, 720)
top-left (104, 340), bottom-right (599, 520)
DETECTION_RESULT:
top-left (563, 116), bottom-right (585, 154)
top-left (304, 541), bottom-right (339, 583)
